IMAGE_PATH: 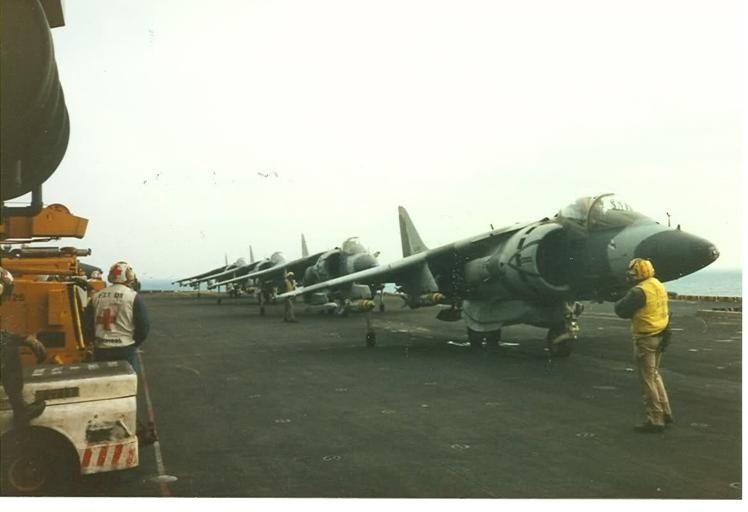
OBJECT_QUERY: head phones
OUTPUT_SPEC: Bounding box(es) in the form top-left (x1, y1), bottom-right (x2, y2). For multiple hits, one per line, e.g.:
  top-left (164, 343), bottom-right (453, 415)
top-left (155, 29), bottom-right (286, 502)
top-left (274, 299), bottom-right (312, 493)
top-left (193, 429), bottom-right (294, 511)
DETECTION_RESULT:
top-left (127, 267), bottom-right (134, 281)
top-left (0, 280), bottom-right (5, 298)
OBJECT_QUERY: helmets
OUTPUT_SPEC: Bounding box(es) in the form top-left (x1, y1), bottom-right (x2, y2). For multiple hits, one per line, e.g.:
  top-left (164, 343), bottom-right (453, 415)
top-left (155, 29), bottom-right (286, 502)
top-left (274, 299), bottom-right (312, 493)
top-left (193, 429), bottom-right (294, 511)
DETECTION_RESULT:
top-left (0, 267), bottom-right (14, 297)
top-left (109, 261), bottom-right (134, 283)
top-left (91, 271), bottom-right (102, 280)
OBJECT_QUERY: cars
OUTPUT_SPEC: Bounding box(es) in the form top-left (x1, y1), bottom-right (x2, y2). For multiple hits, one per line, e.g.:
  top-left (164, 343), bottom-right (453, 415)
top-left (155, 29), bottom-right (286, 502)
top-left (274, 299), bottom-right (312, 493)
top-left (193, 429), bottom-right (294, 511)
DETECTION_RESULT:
top-left (0, 356), bottom-right (139, 499)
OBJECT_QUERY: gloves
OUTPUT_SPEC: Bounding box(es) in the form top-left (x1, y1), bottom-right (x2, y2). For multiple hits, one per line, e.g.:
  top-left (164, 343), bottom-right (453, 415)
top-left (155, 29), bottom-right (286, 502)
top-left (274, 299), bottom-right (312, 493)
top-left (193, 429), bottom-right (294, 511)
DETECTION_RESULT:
top-left (24, 335), bottom-right (48, 364)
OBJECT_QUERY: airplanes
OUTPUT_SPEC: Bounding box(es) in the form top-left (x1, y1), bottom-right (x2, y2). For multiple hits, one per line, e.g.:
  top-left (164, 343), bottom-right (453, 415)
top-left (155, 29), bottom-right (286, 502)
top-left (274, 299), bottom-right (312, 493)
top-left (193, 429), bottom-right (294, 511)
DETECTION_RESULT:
top-left (172, 233), bottom-right (385, 316)
top-left (271, 193), bottom-right (720, 360)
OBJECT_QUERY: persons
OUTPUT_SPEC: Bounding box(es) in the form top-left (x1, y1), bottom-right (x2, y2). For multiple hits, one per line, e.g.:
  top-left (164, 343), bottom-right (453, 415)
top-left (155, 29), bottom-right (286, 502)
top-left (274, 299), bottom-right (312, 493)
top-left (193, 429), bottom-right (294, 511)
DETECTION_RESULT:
top-left (79, 262), bottom-right (153, 448)
top-left (613, 257), bottom-right (674, 434)
top-left (277, 271), bottom-right (299, 323)
top-left (0, 266), bottom-right (48, 427)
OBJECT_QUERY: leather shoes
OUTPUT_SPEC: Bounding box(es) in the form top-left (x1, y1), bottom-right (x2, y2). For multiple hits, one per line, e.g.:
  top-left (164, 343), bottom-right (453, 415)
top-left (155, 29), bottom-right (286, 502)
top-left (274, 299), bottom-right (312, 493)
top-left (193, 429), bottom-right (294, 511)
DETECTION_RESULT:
top-left (13, 400), bottom-right (45, 427)
top-left (666, 419), bottom-right (676, 424)
top-left (635, 422), bottom-right (664, 434)
